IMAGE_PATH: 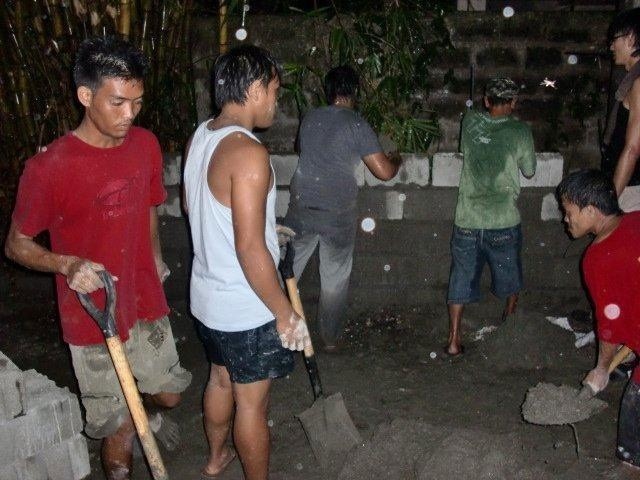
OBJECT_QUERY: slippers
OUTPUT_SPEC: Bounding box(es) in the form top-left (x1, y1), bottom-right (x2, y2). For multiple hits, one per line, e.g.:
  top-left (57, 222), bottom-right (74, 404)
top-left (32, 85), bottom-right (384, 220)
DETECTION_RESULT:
top-left (201, 447), bottom-right (238, 479)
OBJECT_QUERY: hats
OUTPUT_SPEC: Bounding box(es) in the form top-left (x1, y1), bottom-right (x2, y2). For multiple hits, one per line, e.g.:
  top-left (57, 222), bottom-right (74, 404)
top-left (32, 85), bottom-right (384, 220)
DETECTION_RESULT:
top-left (485, 77), bottom-right (519, 99)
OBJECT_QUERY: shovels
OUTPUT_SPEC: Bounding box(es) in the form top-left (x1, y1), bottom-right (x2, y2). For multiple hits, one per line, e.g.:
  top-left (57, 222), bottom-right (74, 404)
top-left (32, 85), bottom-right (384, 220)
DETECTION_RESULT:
top-left (520, 344), bottom-right (632, 424)
top-left (279, 241), bottom-right (364, 466)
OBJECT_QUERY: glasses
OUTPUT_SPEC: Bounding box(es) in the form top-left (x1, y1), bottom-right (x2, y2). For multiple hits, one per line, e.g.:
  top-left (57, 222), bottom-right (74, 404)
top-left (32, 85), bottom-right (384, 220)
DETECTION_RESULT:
top-left (608, 34), bottom-right (630, 46)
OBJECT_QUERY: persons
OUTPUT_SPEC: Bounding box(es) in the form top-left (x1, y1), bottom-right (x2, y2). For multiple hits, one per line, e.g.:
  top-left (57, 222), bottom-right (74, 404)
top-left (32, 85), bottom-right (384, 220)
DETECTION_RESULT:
top-left (443, 69), bottom-right (538, 357)
top-left (549, 163), bottom-right (639, 470)
top-left (275, 60), bottom-right (402, 354)
top-left (4, 28), bottom-right (193, 480)
top-left (606, 3), bottom-right (640, 217)
top-left (182, 42), bottom-right (314, 478)
